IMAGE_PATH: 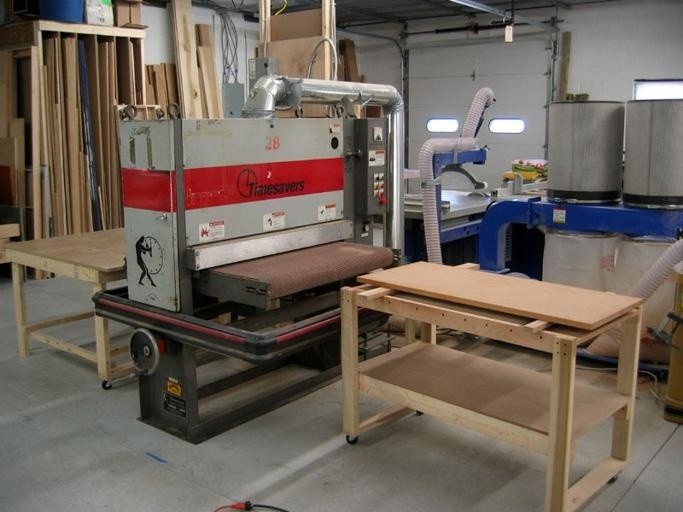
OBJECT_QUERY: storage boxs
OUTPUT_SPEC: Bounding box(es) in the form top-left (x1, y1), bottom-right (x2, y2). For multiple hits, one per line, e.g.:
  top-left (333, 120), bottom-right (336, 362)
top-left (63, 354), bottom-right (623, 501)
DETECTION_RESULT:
top-left (84, 1), bottom-right (148, 29)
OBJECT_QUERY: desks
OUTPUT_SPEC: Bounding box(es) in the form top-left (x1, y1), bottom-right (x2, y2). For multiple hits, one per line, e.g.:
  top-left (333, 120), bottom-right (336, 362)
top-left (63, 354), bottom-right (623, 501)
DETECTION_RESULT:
top-left (2, 228), bottom-right (134, 381)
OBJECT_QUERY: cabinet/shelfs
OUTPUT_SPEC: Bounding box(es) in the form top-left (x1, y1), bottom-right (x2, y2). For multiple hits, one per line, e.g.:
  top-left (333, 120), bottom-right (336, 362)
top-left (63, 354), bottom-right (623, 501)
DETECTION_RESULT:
top-left (336, 262), bottom-right (644, 511)
